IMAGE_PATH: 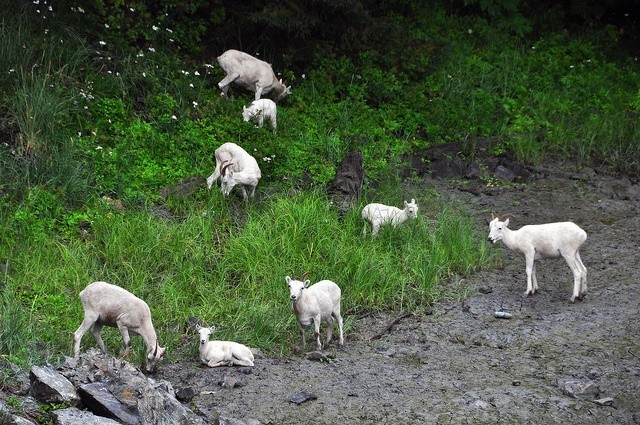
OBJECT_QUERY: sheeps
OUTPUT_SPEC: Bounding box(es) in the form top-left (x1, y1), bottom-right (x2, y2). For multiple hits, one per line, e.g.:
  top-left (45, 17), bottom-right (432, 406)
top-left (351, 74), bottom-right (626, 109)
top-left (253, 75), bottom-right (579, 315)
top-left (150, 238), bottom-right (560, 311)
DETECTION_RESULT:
top-left (206, 141), bottom-right (261, 201)
top-left (485, 211), bottom-right (587, 303)
top-left (285, 269), bottom-right (344, 350)
top-left (196, 323), bottom-right (254, 367)
top-left (242, 98), bottom-right (277, 127)
top-left (73, 281), bottom-right (168, 373)
top-left (217, 48), bottom-right (292, 104)
top-left (362, 197), bottom-right (419, 239)
